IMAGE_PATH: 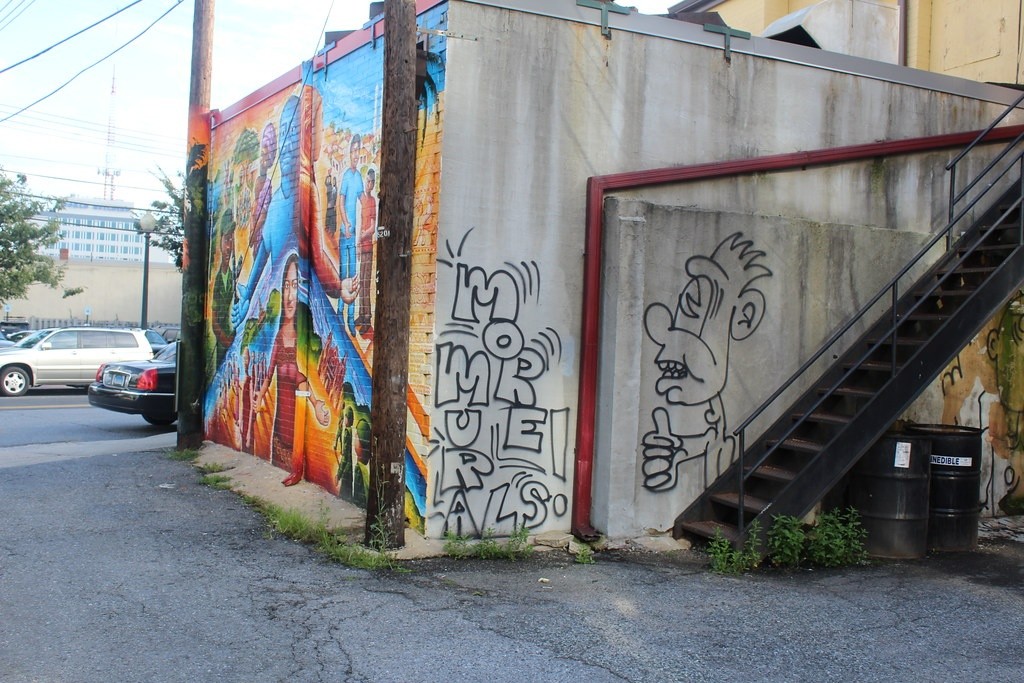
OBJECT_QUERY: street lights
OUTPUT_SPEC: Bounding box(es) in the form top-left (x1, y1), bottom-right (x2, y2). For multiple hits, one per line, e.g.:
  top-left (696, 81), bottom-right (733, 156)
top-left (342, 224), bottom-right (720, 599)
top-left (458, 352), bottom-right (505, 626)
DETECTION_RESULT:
top-left (140, 209), bottom-right (157, 331)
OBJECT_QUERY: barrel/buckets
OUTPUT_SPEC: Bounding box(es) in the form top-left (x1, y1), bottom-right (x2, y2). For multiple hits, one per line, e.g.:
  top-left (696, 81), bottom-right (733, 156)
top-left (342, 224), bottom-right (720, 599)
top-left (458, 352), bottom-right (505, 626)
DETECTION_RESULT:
top-left (903, 423), bottom-right (984, 553)
top-left (850, 431), bottom-right (931, 559)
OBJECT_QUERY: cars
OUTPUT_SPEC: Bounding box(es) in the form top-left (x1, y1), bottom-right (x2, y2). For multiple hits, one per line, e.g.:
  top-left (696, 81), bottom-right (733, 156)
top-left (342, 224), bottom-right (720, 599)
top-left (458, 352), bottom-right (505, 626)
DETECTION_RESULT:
top-left (87, 341), bottom-right (178, 427)
top-left (6, 330), bottom-right (36, 344)
top-left (0, 331), bottom-right (16, 348)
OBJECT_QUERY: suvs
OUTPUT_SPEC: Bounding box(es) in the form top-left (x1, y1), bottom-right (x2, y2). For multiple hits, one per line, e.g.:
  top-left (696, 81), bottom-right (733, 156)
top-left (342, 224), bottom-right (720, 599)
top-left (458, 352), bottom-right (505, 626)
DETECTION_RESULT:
top-left (162, 328), bottom-right (181, 344)
top-left (0, 326), bottom-right (154, 396)
top-left (140, 328), bottom-right (170, 355)
